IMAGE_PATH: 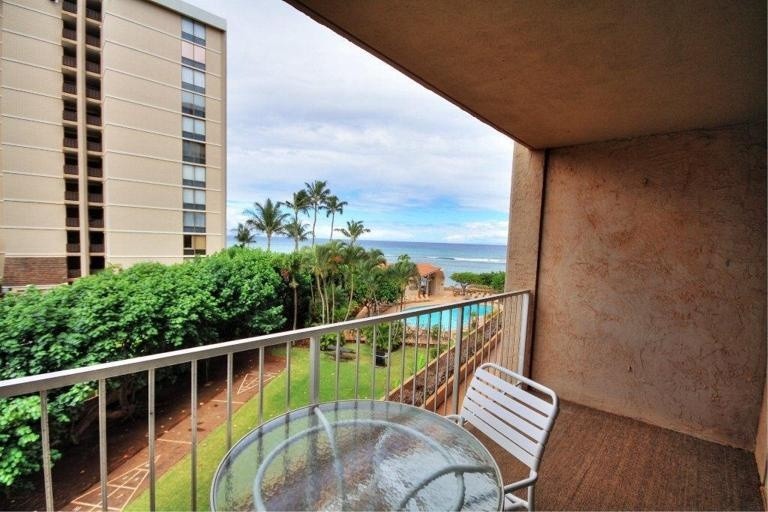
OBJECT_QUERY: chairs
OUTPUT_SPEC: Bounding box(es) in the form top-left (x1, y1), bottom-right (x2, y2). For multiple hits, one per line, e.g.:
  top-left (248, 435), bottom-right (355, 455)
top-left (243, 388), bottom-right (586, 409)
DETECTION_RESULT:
top-left (363, 363), bottom-right (559, 511)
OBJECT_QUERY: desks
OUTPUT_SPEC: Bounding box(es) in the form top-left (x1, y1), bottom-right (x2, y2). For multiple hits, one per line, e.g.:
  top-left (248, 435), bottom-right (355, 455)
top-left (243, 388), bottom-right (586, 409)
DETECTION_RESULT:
top-left (211, 397), bottom-right (505, 512)
